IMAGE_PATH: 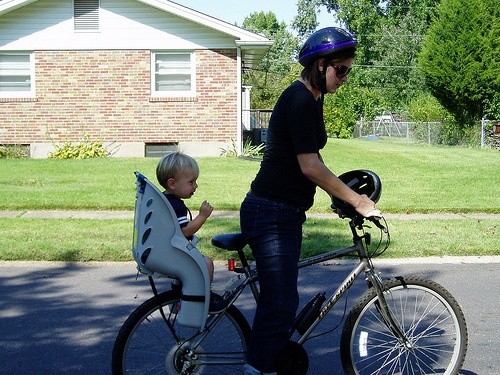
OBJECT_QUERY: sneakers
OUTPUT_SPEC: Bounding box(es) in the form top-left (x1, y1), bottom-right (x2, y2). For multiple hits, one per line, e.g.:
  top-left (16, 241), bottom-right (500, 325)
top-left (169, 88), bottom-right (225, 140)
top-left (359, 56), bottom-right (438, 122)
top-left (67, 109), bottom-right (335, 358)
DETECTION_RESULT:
top-left (169, 302), bottom-right (180, 314)
top-left (209, 292), bottom-right (228, 315)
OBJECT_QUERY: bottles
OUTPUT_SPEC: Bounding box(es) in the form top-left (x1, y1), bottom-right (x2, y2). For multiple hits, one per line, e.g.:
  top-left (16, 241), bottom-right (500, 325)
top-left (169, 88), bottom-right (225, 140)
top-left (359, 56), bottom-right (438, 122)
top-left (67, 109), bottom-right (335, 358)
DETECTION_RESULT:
top-left (294, 291), bottom-right (327, 334)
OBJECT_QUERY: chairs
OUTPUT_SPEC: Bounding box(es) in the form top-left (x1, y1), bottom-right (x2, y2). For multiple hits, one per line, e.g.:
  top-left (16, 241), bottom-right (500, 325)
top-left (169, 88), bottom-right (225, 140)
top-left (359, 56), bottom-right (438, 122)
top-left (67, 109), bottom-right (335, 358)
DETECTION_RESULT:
top-left (131, 170), bottom-right (211, 332)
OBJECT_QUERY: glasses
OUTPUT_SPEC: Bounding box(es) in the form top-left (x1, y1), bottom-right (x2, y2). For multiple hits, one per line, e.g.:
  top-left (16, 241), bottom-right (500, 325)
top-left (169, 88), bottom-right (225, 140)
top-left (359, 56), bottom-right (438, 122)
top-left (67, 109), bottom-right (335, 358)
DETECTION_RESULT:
top-left (330, 62), bottom-right (353, 77)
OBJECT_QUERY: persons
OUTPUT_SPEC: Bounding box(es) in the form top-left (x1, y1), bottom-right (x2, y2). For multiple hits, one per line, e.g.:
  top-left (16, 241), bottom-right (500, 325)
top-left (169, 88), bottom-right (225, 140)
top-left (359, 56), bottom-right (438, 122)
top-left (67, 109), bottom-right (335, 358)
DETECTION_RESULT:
top-left (240, 27), bottom-right (381, 375)
top-left (156, 152), bottom-right (227, 314)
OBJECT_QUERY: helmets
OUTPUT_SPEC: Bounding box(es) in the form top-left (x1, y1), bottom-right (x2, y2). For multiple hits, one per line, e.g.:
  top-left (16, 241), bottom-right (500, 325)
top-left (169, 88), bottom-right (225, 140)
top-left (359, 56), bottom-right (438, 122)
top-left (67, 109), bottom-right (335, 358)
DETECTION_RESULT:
top-left (330, 168), bottom-right (384, 214)
top-left (297, 26), bottom-right (359, 68)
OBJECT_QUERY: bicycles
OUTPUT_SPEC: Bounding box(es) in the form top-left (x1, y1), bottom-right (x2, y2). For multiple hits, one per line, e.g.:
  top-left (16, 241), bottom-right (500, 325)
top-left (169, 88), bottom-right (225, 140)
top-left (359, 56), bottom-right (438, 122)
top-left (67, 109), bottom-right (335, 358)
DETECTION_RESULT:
top-left (112, 194), bottom-right (469, 375)
